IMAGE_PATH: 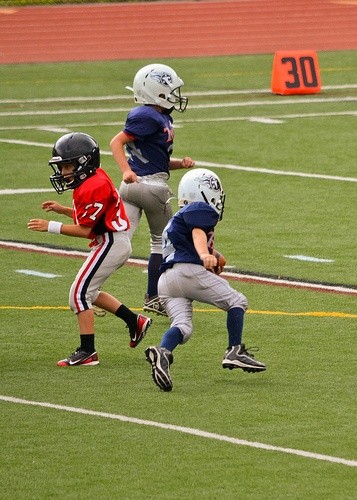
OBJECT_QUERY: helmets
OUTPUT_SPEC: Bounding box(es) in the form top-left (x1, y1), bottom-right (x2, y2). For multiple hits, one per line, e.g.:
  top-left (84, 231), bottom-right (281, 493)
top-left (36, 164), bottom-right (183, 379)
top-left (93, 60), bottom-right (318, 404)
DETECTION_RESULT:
top-left (133, 63), bottom-right (184, 109)
top-left (49, 133), bottom-right (100, 195)
top-left (177, 168), bottom-right (225, 221)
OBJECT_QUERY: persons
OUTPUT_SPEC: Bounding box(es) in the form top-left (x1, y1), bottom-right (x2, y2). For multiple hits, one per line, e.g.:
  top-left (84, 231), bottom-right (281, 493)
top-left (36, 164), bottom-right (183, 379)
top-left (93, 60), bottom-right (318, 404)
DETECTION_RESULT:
top-left (28, 132), bottom-right (152, 366)
top-left (145, 168), bottom-right (266, 392)
top-left (93, 63), bottom-right (194, 317)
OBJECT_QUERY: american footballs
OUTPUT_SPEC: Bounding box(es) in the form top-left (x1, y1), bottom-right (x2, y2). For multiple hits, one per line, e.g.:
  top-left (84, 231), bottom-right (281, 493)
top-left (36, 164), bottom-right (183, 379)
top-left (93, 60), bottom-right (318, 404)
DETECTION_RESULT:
top-left (213, 249), bottom-right (227, 267)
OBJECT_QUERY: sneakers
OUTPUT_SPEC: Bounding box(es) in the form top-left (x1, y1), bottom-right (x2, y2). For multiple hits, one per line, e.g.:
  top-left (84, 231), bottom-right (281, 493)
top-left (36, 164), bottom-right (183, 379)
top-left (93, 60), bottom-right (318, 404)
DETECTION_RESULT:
top-left (143, 293), bottom-right (168, 316)
top-left (126, 314), bottom-right (153, 348)
top-left (145, 346), bottom-right (173, 392)
top-left (221, 344), bottom-right (265, 373)
top-left (56, 347), bottom-right (99, 366)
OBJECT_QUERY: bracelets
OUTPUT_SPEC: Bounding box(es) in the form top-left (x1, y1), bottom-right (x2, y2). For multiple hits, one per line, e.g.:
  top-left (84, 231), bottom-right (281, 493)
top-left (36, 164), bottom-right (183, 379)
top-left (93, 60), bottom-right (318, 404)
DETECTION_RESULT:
top-left (48, 220), bottom-right (63, 234)
